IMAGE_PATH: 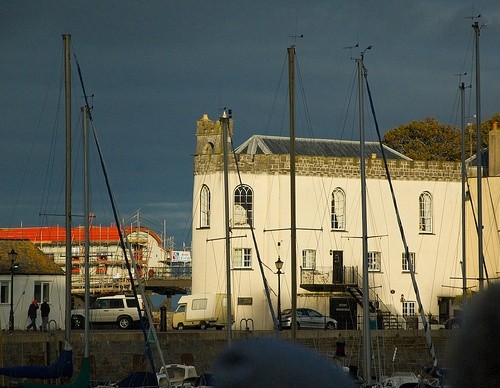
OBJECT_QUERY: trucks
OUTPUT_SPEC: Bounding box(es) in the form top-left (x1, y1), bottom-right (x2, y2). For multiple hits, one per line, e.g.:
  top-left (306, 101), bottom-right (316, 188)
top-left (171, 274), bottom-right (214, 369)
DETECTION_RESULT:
top-left (172, 292), bottom-right (235, 330)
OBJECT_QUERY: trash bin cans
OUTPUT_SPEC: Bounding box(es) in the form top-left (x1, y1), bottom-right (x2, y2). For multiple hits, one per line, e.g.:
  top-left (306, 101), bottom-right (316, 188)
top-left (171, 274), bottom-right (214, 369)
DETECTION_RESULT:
top-left (406, 316), bottom-right (418, 330)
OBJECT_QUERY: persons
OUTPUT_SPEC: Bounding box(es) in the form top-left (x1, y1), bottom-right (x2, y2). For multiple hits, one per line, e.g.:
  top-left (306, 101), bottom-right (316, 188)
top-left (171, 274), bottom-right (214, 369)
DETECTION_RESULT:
top-left (212, 338), bottom-right (355, 388)
top-left (27, 301), bottom-right (39, 331)
top-left (40, 297), bottom-right (50, 332)
top-left (446, 280), bottom-right (500, 388)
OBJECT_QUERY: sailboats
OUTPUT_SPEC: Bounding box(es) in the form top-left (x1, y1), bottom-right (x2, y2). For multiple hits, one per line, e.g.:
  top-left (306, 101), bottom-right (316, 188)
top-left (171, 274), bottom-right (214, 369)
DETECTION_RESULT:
top-left (0, 0), bottom-right (500, 388)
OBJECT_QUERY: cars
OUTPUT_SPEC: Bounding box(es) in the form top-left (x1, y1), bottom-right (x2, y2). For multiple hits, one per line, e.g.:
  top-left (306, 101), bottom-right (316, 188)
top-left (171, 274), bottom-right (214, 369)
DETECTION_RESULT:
top-left (277, 308), bottom-right (338, 330)
top-left (445, 316), bottom-right (464, 330)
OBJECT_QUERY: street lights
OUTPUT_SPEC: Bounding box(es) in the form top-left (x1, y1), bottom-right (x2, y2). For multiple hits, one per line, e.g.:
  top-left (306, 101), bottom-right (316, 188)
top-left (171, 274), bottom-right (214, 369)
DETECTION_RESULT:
top-left (8, 248), bottom-right (18, 334)
top-left (275, 257), bottom-right (284, 331)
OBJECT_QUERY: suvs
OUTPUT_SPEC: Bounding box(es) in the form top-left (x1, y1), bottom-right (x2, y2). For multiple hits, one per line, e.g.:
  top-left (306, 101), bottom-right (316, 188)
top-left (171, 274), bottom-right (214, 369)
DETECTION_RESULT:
top-left (71, 294), bottom-right (154, 330)
